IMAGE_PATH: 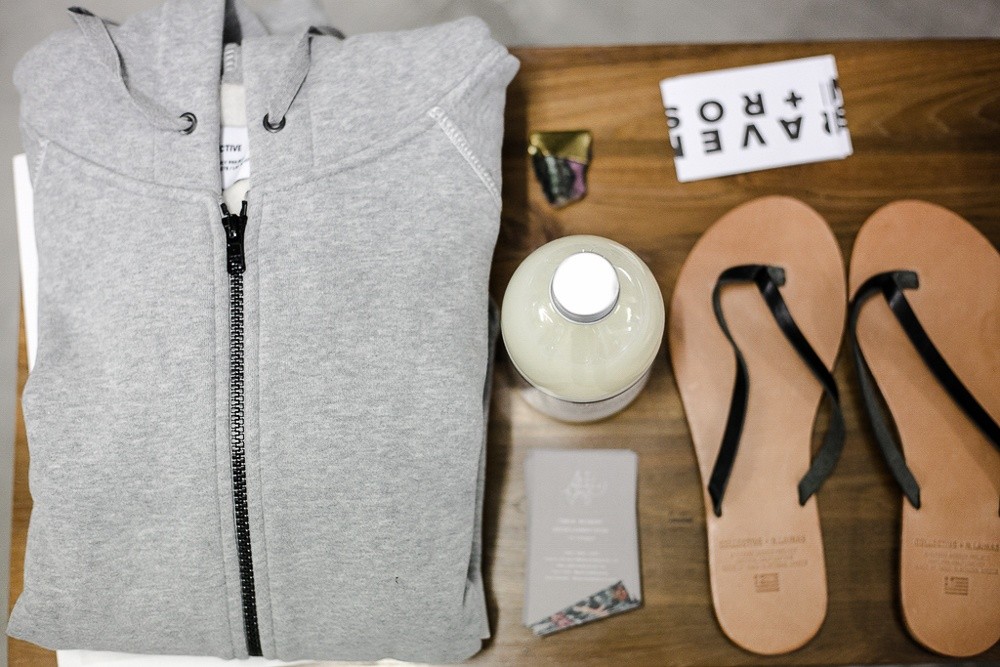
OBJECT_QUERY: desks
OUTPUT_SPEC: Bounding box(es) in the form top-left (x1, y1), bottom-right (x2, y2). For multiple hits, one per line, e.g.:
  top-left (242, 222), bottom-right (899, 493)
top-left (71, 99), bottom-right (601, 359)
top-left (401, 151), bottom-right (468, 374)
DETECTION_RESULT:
top-left (5, 38), bottom-right (1000, 667)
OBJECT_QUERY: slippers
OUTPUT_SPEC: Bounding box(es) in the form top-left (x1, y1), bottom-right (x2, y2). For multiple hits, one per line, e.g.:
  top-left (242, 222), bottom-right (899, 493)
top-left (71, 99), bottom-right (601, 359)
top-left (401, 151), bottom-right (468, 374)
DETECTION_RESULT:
top-left (667, 195), bottom-right (841, 652)
top-left (848, 199), bottom-right (1000, 657)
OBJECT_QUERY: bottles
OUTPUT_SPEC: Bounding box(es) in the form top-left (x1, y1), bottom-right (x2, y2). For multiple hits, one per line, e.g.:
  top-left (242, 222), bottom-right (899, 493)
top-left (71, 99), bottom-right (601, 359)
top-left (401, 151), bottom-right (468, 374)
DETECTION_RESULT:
top-left (501, 235), bottom-right (665, 423)
